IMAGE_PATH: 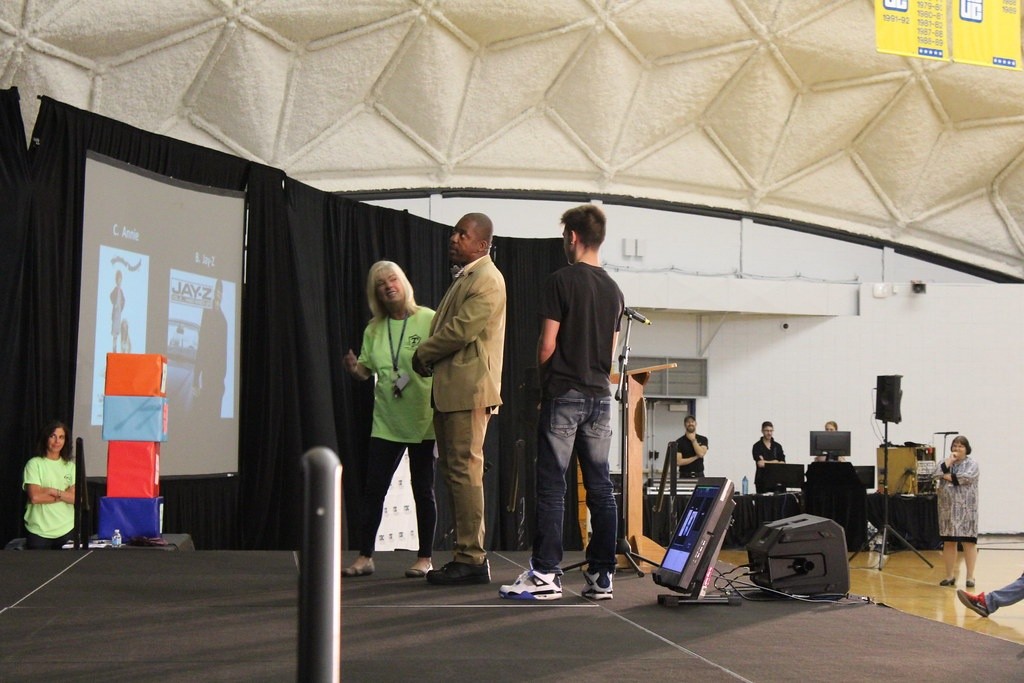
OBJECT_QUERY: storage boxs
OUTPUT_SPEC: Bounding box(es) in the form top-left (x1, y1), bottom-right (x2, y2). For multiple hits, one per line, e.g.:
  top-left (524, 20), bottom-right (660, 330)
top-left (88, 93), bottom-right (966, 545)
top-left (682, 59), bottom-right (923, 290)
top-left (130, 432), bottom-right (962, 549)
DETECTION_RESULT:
top-left (99, 352), bottom-right (168, 541)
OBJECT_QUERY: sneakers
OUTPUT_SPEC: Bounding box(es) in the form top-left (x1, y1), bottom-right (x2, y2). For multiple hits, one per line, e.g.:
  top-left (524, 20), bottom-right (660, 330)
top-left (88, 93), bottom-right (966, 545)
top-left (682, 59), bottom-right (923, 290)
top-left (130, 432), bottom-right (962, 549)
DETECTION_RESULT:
top-left (498, 556), bottom-right (562, 601)
top-left (957, 590), bottom-right (990, 617)
top-left (581, 571), bottom-right (614, 600)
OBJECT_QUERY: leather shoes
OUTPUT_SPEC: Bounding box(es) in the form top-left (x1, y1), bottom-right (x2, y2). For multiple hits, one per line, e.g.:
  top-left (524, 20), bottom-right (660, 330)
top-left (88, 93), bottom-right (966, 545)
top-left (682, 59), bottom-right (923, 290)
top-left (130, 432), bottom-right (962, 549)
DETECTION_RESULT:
top-left (426, 559), bottom-right (491, 585)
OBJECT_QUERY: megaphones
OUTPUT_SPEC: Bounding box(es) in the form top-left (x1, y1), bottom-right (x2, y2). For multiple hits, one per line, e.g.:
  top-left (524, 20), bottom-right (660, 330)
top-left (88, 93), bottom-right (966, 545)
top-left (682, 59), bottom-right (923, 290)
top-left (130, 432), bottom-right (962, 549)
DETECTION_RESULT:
top-left (911, 283), bottom-right (926, 294)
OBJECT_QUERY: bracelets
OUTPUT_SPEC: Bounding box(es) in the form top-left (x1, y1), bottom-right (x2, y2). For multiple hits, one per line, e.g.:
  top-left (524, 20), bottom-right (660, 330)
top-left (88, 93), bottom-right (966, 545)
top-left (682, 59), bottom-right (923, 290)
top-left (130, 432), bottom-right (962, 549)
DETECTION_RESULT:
top-left (57, 489), bottom-right (62, 499)
top-left (691, 439), bottom-right (697, 442)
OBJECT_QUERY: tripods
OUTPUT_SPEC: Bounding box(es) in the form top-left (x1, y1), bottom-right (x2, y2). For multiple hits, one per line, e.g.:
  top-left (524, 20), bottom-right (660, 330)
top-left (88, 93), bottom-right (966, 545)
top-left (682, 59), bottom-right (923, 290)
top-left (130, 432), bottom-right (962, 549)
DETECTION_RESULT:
top-left (849, 421), bottom-right (934, 571)
top-left (560, 315), bottom-right (661, 578)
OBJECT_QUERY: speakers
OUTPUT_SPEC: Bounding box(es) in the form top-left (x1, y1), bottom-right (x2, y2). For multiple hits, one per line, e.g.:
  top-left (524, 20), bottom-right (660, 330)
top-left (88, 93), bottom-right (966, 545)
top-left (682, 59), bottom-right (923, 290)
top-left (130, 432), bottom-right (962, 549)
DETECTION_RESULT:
top-left (747, 513), bottom-right (851, 601)
top-left (875, 375), bottom-right (903, 424)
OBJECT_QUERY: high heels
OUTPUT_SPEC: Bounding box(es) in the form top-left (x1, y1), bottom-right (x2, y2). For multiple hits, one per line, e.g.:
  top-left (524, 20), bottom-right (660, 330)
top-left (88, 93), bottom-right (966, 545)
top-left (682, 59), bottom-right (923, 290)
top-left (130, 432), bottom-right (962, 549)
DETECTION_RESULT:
top-left (941, 579), bottom-right (955, 586)
top-left (966, 579), bottom-right (975, 587)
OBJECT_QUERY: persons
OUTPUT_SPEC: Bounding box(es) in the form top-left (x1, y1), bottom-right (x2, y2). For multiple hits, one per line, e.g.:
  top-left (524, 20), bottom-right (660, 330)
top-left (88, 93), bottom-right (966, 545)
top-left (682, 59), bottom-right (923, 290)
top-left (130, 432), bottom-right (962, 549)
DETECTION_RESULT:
top-left (109, 272), bottom-right (126, 354)
top-left (192, 279), bottom-right (228, 418)
top-left (22, 421), bottom-right (76, 551)
top-left (957, 572), bottom-right (1024, 617)
top-left (676, 415), bottom-right (708, 478)
top-left (752, 421), bottom-right (785, 496)
top-left (930, 436), bottom-right (978, 587)
top-left (497, 204), bottom-right (625, 602)
top-left (339, 260), bottom-right (438, 578)
top-left (815, 421), bottom-right (847, 462)
top-left (412, 212), bottom-right (506, 585)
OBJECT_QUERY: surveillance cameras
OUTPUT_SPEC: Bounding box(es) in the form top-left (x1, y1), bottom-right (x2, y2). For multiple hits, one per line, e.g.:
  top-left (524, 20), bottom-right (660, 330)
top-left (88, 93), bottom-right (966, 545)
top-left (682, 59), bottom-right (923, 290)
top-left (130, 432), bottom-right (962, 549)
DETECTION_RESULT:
top-left (782, 321), bottom-right (790, 330)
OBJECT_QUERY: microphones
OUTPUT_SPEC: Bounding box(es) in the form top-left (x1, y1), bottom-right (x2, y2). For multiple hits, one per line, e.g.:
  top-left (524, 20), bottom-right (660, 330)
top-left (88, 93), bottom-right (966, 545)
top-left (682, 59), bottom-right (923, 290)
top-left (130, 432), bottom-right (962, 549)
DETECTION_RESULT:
top-left (624, 307), bottom-right (652, 325)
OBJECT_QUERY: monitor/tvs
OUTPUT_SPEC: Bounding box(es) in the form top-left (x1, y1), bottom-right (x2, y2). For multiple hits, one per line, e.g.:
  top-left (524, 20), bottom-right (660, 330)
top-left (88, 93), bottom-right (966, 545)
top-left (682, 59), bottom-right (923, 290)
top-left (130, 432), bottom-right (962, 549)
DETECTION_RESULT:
top-left (854, 465), bottom-right (875, 489)
top-left (810, 431), bottom-right (851, 462)
top-left (652, 475), bottom-right (735, 594)
top-left (762, 463), bottom-right (804, 495)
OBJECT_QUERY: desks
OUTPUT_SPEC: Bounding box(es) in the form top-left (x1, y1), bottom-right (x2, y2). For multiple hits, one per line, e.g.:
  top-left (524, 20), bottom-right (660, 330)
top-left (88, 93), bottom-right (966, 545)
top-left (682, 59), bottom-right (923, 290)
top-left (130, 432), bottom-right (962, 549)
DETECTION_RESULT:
top-left (614, 492), bottom-right (944, 551)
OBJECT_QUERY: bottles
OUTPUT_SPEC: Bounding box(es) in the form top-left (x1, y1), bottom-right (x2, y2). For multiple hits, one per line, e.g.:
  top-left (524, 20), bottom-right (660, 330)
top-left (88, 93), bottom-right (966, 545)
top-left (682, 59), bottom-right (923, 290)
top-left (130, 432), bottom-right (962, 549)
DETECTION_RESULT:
top-left (112, 529), bottom-right (122, 548)
top-left (742, 476), bottom-right (748, 495)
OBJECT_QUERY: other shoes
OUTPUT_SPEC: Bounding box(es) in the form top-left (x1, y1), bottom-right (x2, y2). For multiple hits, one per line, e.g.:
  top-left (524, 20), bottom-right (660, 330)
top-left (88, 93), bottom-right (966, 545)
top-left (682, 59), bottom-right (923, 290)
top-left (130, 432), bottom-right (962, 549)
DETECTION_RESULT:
top-left (343, 564), bottom-right (374, 576)
top-left (405, 562), bottom-right (433, 577)
